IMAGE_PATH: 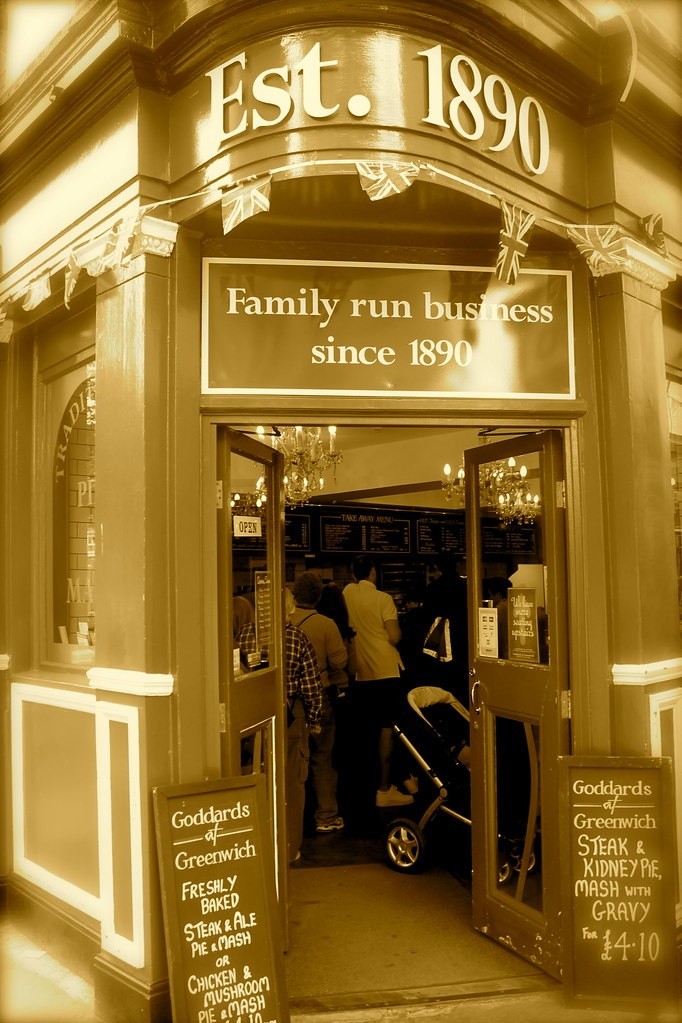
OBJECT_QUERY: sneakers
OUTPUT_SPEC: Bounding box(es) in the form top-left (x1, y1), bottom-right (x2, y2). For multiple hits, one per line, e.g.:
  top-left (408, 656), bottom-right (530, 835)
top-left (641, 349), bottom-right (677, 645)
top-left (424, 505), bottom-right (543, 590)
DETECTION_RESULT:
top-left (317, 817), bottom-right (345, 831)
top-left (406, 774), bottom-right (419, 793)
top-left (375, 785), bottom-right (414, 806)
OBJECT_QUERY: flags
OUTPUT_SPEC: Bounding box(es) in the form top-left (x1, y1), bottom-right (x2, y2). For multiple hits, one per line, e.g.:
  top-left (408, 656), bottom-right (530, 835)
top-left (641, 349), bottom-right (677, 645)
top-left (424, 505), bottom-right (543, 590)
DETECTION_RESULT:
top-left (356, 161), bottom-right (422, 201)
top-left (449, 269), bottom-right (493, 319)
top-left (103, 206), bottom-right (149, 272)
top-left (567, 226), bottom-right (632, 270)
top-left (219, 172), bottom-right (272, 236)
top-left (0, 298), bottom-right (14, 346)
top-left (638, 212), bottom-right (668, 255)
top-left (22, 269), bottom-right (53, 311)
top-left (61, 252), bottom-right (83, 310)
top-left (313, 266), bottom-right (354, 317)
top-left (496, 201), bottom-right (535, 284)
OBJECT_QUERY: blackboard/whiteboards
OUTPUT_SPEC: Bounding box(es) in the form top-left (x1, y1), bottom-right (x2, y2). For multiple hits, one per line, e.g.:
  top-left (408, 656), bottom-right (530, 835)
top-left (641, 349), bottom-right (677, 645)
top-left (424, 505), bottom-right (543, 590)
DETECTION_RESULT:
top-left (151, 773), bottom-right (289, 1023)
top-left (556, 755), bottom-right (679, 1014)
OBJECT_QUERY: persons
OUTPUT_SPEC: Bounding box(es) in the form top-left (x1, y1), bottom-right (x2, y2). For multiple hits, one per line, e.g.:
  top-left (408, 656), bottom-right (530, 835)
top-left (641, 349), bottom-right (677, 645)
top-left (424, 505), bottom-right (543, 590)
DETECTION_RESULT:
top-left (484, 575), bottom-right (515, 658)
top-left (287, 571), bottom-right (348, 833)
top-left (234, 586), bottom-right (322, 863)
top-left (414, 550), bottom-right (469, 706)
top-left (343, 556), bottom-right (415, 807)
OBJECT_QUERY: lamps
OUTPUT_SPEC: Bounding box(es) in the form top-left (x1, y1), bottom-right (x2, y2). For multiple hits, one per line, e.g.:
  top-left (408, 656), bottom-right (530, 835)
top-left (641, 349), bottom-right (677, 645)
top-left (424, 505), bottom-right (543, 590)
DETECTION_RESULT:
top-left (272, 425), bottom-right (344, 511)
top-left (441, 432), bottom-right (528, 508)
top-left (230, 475), bottom-right (272, 528)
top-left (496, 466), bottom-right (542, 527)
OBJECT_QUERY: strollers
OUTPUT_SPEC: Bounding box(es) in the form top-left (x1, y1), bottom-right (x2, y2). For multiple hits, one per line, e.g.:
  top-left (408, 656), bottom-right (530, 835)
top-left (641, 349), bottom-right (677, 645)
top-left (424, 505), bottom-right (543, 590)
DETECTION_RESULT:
top-left (381, 672), bottom-right (539, 885)
